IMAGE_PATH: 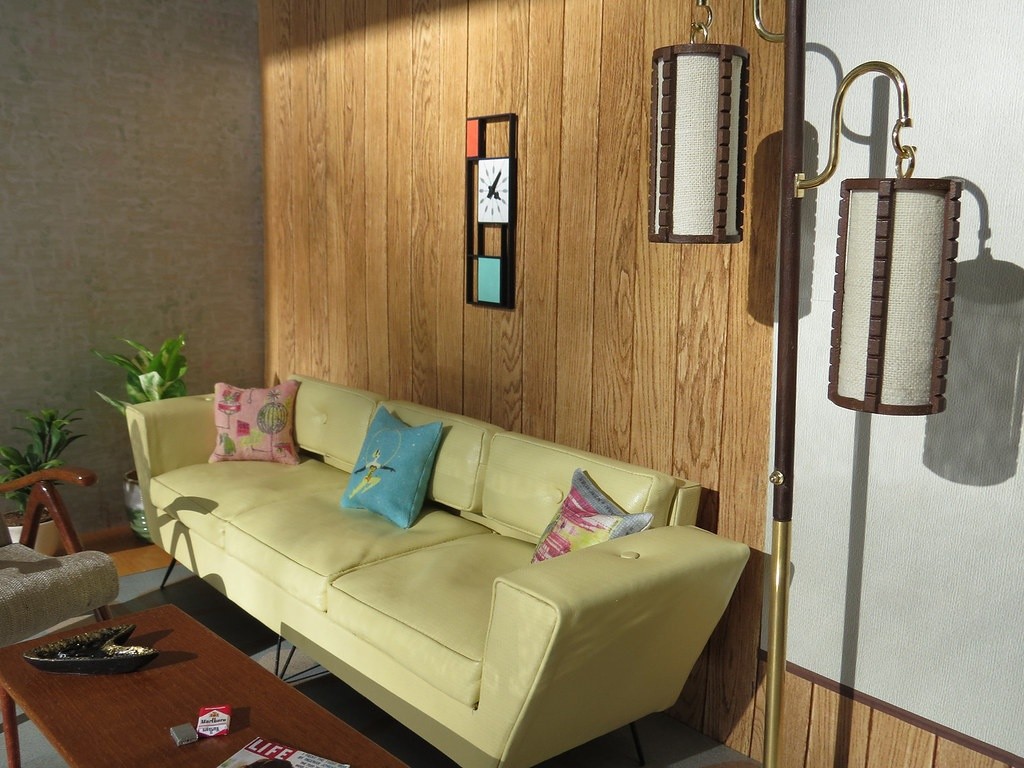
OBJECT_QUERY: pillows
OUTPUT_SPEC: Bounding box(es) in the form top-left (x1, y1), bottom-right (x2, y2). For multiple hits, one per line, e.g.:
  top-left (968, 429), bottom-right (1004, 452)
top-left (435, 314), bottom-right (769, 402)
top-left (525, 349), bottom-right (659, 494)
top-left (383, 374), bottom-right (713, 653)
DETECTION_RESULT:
top-left (339, 406), bottom-right (442, 529)
top-left (207, 380), bottom-right (304, 469)
top-left (531, 466), bottom-right (654, 563)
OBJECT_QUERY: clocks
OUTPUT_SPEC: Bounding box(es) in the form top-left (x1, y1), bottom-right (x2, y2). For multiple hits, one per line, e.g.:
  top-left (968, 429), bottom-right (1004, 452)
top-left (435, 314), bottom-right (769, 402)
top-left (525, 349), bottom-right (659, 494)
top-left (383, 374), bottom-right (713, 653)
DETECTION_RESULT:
top-left (477, 158), bottom-right (510, 223)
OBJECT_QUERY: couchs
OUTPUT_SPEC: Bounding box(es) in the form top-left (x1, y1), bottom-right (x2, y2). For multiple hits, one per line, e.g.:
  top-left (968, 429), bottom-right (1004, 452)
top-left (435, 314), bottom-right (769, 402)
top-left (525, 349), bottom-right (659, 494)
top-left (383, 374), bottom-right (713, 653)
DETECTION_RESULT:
top-left (126, 373), bottom-right (753, 768)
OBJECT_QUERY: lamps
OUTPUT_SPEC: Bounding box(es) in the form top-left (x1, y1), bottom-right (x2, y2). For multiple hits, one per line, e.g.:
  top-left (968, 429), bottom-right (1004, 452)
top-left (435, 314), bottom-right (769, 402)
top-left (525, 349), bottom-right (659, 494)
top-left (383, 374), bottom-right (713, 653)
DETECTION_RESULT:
top-left (637, 0), bottom-right (965, 768)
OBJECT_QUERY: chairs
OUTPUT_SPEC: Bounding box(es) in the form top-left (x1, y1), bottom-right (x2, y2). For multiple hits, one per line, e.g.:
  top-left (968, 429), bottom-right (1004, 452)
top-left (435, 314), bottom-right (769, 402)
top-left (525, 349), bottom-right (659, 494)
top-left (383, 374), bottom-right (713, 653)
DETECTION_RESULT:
top-left (0, 464), bottom-right (119, 648)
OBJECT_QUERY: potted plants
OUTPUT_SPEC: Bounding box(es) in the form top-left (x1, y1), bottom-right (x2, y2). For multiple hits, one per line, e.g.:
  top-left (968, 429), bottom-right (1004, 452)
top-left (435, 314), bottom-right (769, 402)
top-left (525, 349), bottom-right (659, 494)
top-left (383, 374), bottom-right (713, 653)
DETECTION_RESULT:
top-left (0, 402), bottom-right (88, 559)
top-left (91, 326), bottom-right (190, 549)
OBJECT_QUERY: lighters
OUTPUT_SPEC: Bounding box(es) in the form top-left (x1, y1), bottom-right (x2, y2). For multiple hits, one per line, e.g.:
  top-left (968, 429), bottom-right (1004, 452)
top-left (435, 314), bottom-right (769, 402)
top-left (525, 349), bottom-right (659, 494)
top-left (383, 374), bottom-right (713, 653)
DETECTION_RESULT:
top-left (168, 722), bottom-right (200, 746)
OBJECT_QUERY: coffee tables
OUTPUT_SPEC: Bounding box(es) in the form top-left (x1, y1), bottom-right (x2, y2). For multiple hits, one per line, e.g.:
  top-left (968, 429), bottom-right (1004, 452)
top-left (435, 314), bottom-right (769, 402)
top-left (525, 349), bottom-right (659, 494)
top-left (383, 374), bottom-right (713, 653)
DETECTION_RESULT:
top-left (0, 604), bottom-right (410, 768)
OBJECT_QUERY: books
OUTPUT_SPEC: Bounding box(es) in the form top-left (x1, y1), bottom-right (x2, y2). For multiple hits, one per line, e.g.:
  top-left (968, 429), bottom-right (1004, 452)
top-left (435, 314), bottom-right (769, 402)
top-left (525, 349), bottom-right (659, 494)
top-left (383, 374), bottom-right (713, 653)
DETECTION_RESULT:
top-left (212, 738), bottom-right (350, 767)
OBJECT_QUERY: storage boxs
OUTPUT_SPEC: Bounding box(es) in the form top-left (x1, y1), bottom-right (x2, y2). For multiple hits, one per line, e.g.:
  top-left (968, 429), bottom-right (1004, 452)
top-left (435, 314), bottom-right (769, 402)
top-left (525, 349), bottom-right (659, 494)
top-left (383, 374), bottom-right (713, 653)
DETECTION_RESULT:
top-left (197, 704), bottom-right (232, 737)
